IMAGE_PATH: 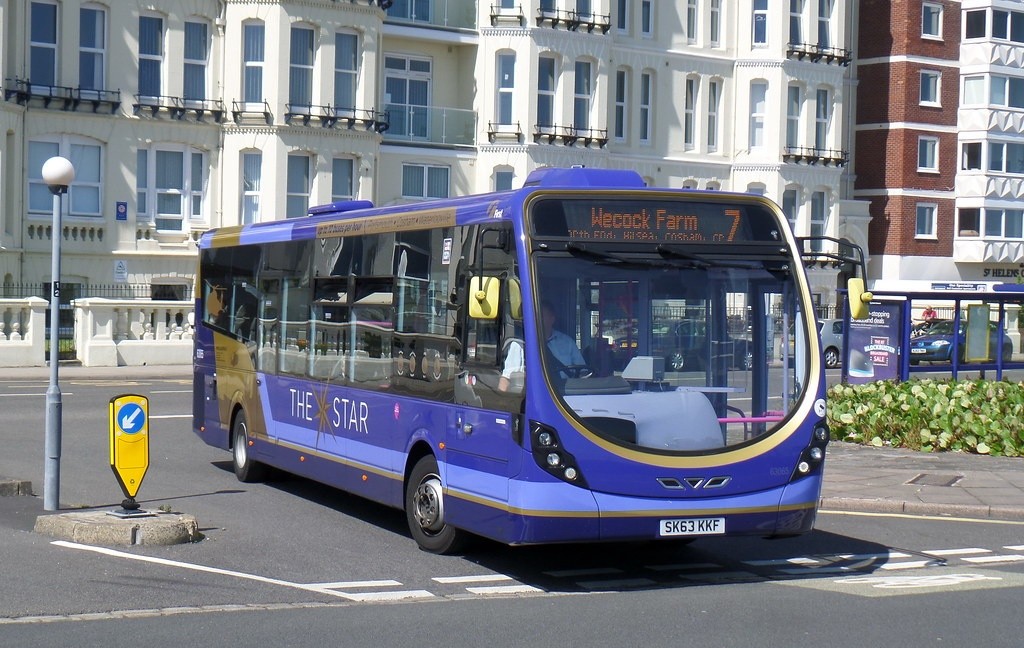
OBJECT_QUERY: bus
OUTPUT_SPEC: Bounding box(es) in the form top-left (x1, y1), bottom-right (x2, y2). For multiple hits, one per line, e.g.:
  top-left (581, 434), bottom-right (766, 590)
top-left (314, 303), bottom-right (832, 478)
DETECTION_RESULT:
top-left (191, 167), bottom-right (873, 556)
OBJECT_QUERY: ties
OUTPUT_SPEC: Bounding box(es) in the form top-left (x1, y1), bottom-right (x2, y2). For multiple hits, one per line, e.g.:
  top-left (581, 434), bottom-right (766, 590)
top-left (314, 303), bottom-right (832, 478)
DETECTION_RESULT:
top-left (546, 345), bottom-right (561, 384)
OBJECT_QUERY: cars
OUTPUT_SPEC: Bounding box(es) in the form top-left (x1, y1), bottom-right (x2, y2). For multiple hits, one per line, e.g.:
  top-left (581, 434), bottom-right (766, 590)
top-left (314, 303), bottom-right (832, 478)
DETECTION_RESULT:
top-left (611, 318), bottom-right (757, 373)
top-left (907, 319), bottom-right (1015, 365)
top-left (779, 319), bottom-right (845, 368)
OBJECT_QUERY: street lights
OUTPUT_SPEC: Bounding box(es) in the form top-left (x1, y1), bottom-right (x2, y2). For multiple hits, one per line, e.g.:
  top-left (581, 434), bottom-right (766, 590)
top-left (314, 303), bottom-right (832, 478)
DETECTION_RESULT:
top-left (41, 157), bottom-right (77, 512)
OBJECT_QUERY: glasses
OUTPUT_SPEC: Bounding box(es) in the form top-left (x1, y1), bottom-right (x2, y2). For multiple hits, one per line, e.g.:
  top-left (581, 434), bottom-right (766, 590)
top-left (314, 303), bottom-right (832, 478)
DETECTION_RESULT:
top-left (926, 309), bottom-right (931, 310)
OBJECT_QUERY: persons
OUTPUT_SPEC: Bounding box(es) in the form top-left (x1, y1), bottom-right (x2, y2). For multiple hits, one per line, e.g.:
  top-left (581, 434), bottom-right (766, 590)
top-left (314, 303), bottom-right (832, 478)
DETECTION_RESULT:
top-left (494, 301), bottom-right (589, 393)
top-left (922, 305), bottom-right (937, 322)
